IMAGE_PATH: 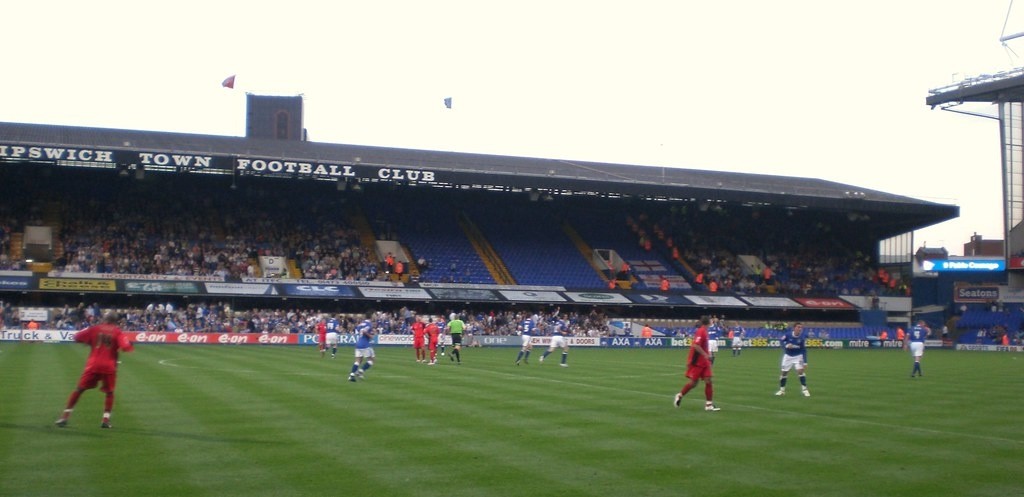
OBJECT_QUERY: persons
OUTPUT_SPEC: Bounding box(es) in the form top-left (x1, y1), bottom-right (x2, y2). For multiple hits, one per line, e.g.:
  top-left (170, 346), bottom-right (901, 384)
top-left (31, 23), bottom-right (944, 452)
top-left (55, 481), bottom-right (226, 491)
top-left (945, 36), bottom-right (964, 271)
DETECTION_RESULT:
top-left (776, 322), bottom-right (810, 397)
top-left (880, 327), bottom-right (906, 348)
top-left (0, 194), bottom-right (427, 284)
top-left (540, 313), bottom-right (570, 367)
top-left (348, 310), bottom-right (377, 382)
top-left (903, 319), bottom-right (927, 378)
top-left (708, 314), bottom-right (745, 364)
top-left (925, 297), bottom-right (1024, 346)
top-left (642, 324), bottom-right (652, 338)
top-left (315, 312), bottom-right (341, 359)
top-left (674, 314), bottom-right (721, 411)
top-left (441, 261), bottom-right (471, 283)
top-left (673, 327), bottom-right (690, 337)
top-left (515, 314), bottom-right (536, 365)
top-left (607, 203), bottom-right (911, 296)
top-left (54, 310), bottom-right (133, 429)
top-left (756, 321), bottom-right (788, 338)
top-left (808, 328), bottom-right (830, 338)
top-left (0, 300), bottom-right (631, 336)
top-left (410, 314), bottom-right (482, 365)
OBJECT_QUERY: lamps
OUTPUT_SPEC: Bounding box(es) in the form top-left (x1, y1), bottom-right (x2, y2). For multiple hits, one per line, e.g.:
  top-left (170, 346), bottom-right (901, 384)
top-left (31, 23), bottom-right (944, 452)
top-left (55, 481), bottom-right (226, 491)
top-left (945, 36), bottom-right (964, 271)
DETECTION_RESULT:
top-left (542, 193), bottom-right (553, 200)
top-left (119, 164), bottom-right (128, 175)
top-left (848, 212), bottom-right (870, 222)
top-left (353, 184), bottom-right (360, 189)
top-left (713, 202), bottom-right (722, 210)
top-left (786, 210), bottom-right (793, 215)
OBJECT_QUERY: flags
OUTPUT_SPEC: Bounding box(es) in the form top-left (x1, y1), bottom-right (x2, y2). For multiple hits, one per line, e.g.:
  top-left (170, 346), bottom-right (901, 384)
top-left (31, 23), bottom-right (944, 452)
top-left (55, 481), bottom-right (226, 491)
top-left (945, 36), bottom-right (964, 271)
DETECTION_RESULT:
top-left (222, 75), bottom-right (235, 89)
top-left (444, 98), bottom-right (452, 108)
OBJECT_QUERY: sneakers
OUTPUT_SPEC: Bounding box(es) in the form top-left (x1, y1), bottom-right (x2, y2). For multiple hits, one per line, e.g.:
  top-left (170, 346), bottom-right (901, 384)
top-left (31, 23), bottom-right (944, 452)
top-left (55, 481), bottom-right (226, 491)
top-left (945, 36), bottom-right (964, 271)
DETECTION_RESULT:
top-left (802, 388), bottom-right (811, 397)
top-left (705, 403), bottom-right (720, 411)
top-left (674, 392), bottom-right (683, 408)
top-left (348, 376), bottom-right (356, 382)
top-left (101, 422), bottom-right (113, 428)
top-left (775, 390), bottom-right (786, 396)
top-left (56, 419), bottom-right (67, 426)
top-left (355, 370), bottom-right (364, 380)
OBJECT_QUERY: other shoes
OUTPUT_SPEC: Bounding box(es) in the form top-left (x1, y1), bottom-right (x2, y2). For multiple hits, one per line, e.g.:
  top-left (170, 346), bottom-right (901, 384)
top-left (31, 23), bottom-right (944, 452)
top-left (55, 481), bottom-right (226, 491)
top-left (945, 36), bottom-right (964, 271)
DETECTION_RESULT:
top-left (540, 356), bottom-right (544, 363)
top-left (515, 361), bottom-right (520, 366)
top-left (330, 354), bottom-right (334, 359)
top-left (449, 353), bottom-right (454, 361)
top-left (428, 362), bottom-right (434, 365)
top-left (524, 359), bottom-right (528, 364)
top-left (561, 364), bottom-right (568, 368)
top-left (441, 353), bottom-right (444, 355)
top-left (458, 360), bottom-right (461, 363)
top-left (422, 360), bottom-right (427, 362)
top-left (416, 360), bottom-right (420, 362)
top-left (434, 359), bottom-right (437, 363)
